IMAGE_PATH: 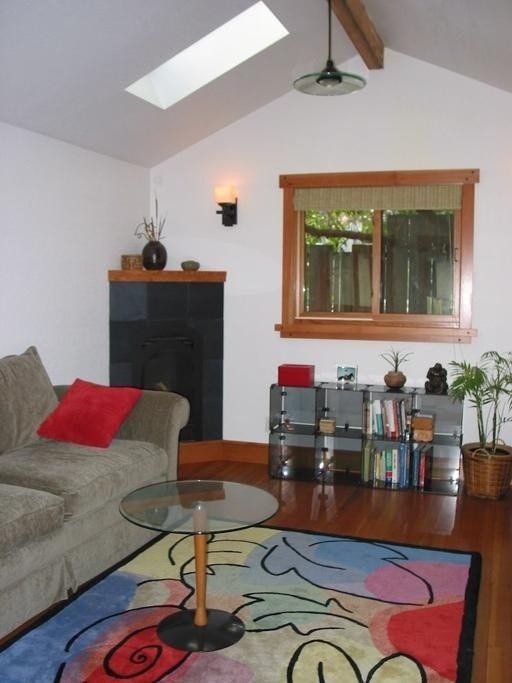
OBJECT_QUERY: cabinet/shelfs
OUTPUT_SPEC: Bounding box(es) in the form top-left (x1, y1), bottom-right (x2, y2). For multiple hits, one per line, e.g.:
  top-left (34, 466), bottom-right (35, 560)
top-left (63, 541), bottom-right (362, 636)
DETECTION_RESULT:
top-left (269, 380), bottom-right (464, 496)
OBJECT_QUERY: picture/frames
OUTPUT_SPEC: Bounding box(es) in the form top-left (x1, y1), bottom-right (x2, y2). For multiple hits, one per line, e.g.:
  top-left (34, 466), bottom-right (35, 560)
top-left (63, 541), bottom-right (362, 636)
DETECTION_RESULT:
top-left (336, 365), bottom-right (358, 384)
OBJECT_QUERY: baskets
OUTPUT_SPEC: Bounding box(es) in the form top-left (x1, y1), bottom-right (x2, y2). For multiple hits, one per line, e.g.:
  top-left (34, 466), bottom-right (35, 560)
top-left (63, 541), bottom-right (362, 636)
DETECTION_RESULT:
top-left (462, 441), bottom-right (512, 499)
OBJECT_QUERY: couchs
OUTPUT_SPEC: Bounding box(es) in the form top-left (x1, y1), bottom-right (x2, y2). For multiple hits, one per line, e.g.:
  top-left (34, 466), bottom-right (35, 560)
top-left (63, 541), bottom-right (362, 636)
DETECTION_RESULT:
top-left (0, 346), bottom-right (191, 641)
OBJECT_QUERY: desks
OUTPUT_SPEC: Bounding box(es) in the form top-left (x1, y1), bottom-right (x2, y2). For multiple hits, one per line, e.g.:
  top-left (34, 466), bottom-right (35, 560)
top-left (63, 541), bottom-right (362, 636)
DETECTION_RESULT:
top-left (120, 480), bottom-right (279, 652)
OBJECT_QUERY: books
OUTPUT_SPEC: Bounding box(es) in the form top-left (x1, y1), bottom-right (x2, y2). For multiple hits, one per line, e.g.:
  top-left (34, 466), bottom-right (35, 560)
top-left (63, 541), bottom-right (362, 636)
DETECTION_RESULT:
top-left (363, 398), bottom-right (432, 491)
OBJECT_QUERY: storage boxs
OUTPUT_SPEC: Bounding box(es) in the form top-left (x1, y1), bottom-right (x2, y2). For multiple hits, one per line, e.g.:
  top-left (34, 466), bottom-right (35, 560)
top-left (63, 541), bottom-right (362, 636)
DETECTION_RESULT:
top-left (278, 363), bottom-right (315, 386)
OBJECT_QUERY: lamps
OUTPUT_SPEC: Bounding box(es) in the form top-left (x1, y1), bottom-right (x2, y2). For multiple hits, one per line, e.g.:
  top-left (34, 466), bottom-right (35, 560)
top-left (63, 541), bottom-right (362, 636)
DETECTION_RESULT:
top-left (216, 184), bottom-right (238, 227)
top-left (293, 0), bottom-right (365, 96)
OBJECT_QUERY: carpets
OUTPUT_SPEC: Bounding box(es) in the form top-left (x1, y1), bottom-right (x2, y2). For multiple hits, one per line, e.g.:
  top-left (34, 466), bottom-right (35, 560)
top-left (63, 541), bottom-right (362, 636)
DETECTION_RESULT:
top-left (0, 515), bottom-right (481, 683)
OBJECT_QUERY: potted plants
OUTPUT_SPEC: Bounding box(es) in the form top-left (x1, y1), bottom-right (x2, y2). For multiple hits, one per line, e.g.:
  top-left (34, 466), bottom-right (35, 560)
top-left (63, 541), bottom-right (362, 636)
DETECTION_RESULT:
top-left (381, 345), bottom-right (413, 388)
top-left (134, 196), bottom-right (168, 271)
top-left (449, 352), bottom-right (512, 500)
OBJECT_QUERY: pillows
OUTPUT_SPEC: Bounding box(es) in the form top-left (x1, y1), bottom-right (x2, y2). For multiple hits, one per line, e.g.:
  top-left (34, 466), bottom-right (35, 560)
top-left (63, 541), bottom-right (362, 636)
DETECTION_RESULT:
top-left (0, 346), bottom-right (60, 457)
top-left (37, 379), bottom-right (145, 447)
